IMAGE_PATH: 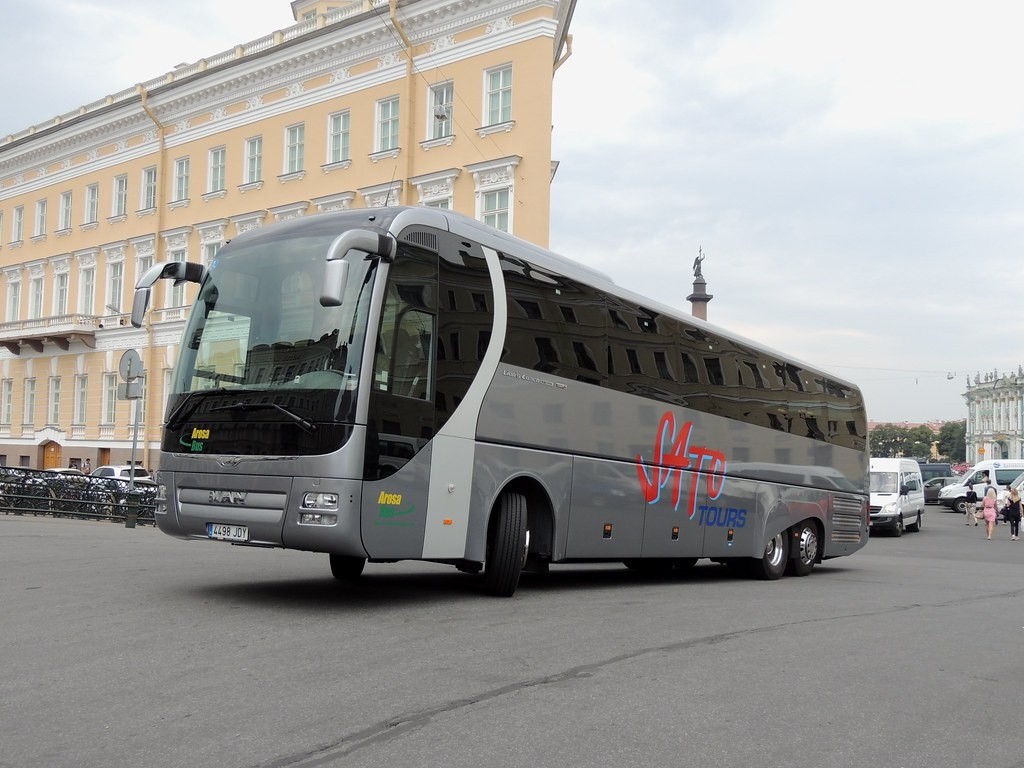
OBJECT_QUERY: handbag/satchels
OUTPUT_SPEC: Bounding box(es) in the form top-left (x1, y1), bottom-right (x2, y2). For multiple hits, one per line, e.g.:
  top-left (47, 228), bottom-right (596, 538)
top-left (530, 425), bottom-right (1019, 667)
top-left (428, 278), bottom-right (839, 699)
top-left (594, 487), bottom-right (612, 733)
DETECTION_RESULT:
top-left (964, 502), bottom-right (971, 508)
top-left (975, 506), bottom-right (983, 518)
top-left (998, 503), bottom-right (1009, 515)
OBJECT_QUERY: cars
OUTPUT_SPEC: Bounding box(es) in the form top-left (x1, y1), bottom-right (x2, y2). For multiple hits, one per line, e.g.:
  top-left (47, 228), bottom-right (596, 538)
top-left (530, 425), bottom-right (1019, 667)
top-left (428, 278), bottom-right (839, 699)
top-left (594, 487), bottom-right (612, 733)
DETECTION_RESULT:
top-left (0, 465), bottom-right (49, 494)
top-left (85, 466), bottom-right (158, 503)
top-left (922, 476), bottom-right (962, 504)
top-left (43, 467), bottom-right (90, 495)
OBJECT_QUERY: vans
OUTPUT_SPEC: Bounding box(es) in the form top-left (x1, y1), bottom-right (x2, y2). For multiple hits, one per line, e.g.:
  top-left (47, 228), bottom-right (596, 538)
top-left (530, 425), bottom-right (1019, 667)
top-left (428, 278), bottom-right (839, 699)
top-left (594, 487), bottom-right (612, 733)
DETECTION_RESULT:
top-left (919, 462), bottom-right (951, 483)
top-left (995, 470), bottom-right (1024, 519)
top-left (937, 458), bottom-right (1024, 513)
top-left (869, 458), bottom-right (925, 537)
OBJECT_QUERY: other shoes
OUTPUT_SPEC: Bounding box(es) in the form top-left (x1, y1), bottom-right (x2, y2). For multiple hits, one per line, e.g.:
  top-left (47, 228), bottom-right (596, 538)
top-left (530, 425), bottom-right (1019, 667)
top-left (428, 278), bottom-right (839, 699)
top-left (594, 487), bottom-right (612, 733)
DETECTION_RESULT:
top-left (974, 522), bottom-right (978, 526)
top-left (965, 523), bottom-right (969, 525)
top-left (1015, 536), bottom-right (1020, 541)
top-left (1002, 522), bottom-right (1007, 526)
top-left (1011, 535), bottom-right (1015, 541)
top-left (987, 537), bottom-right (991, 540)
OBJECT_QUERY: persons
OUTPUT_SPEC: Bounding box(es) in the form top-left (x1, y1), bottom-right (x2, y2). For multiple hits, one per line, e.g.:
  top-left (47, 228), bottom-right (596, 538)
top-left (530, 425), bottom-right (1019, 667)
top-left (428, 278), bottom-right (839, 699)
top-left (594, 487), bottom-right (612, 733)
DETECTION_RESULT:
top-left (964, 485), bottom-right (978, 526)
top-left (1007, 488), bottom-right (1023, 541)
top-left (982, 488), bottom-right (996, 540)
top-left (81, 458), bottom-right (91, 474)
top-left (984, 479), bottom-right (999, 525)
top-left (148, 469), bottom-right (155, 482)
top-left (1002, 486), bottom-right (1012, 526)
top-left (69, 461), bottom-right (77, 469)
top-left (980, 472), bottom-right (988, 483)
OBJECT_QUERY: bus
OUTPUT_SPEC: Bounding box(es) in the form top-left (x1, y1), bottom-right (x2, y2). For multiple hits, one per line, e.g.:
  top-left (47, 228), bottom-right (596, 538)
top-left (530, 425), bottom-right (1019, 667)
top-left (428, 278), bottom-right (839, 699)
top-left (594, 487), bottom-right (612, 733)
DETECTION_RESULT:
top-left (130, 203), bottom-right (871, 597)
